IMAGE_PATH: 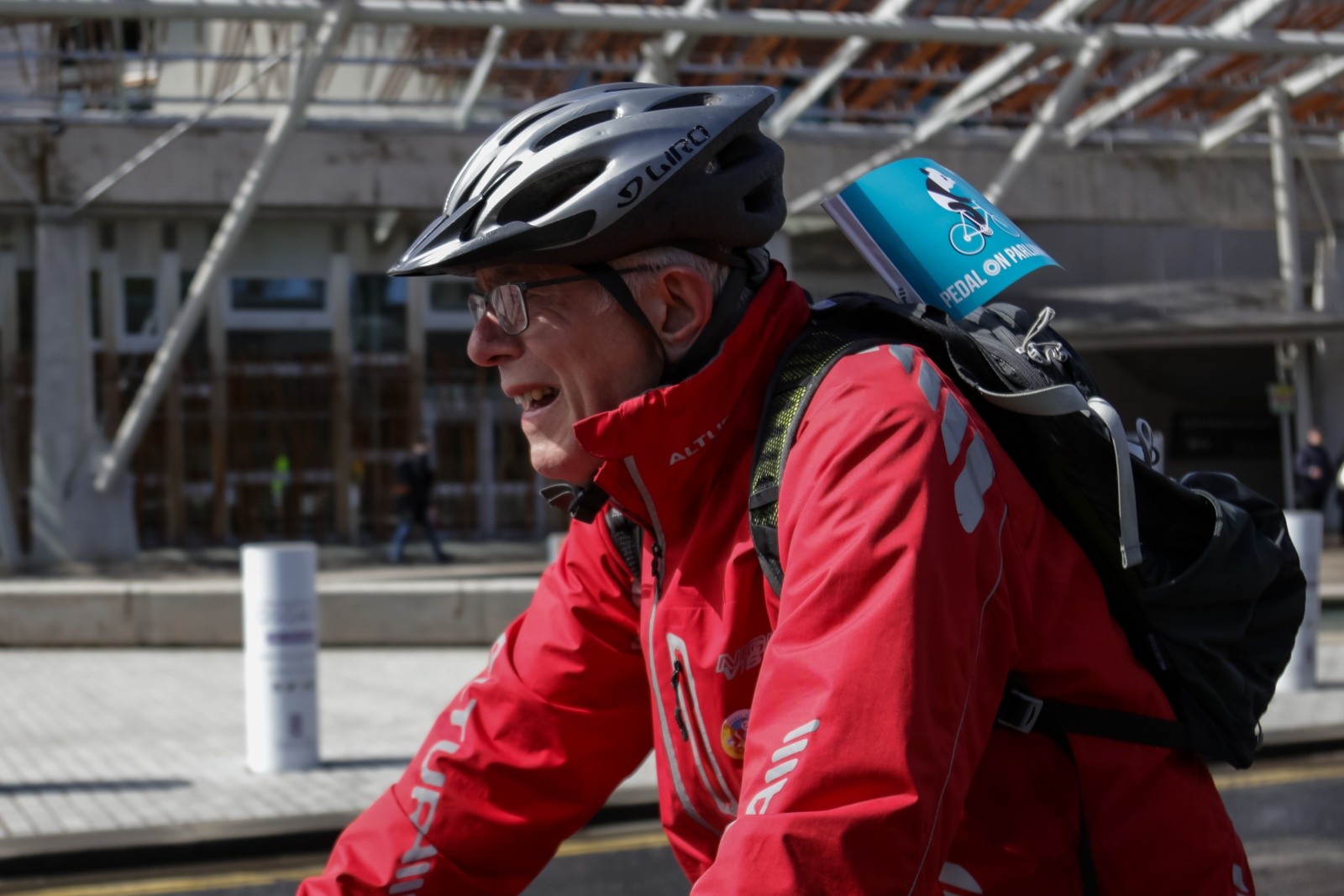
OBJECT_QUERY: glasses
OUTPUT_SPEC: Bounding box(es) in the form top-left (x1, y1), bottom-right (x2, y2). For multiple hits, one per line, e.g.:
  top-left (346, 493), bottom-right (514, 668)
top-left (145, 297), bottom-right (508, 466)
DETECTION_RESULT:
top-left (467, 265), bottom-right (662, 334)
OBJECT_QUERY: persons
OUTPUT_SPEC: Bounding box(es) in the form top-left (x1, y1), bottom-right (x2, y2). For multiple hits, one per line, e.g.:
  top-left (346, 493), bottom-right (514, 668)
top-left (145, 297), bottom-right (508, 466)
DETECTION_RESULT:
top-left (386, 437), bottom-right (455, 565)
top-left (295, 82), bottom-right (1259, 896)
top-left (1296, 427), bottom-right (1334, 510)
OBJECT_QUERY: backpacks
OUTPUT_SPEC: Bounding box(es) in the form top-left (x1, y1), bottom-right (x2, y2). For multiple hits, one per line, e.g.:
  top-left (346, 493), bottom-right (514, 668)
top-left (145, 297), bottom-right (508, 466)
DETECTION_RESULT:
top-left (605, 293), bottom-right (1308, 769)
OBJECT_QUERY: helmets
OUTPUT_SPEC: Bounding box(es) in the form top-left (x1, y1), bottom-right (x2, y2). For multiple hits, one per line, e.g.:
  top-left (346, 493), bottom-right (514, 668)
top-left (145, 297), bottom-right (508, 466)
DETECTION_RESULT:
top-left (386, 82), bottom-right (787, 278)
